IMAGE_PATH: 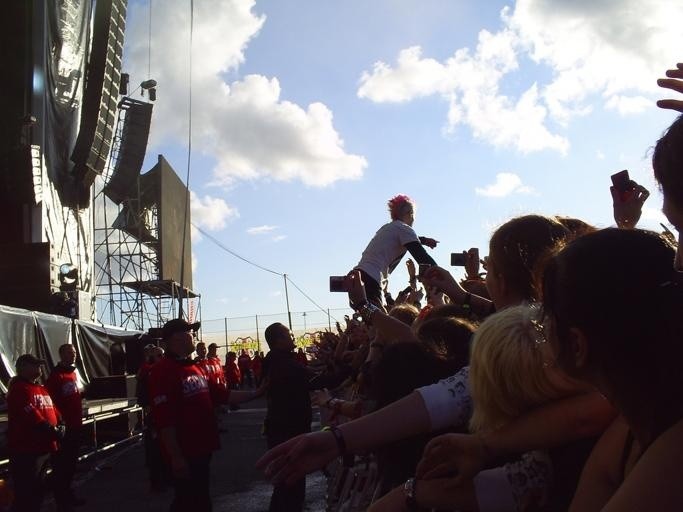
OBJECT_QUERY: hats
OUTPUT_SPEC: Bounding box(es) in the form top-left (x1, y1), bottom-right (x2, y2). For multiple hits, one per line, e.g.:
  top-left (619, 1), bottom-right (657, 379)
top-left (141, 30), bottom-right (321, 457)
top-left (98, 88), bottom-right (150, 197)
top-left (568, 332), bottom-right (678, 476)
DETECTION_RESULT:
top-left (162, 319), bottom-right (201, 338)
top-left (15, 354), bottom-right (46, 367)
top-left (208, 343), bottom-right (221, 348)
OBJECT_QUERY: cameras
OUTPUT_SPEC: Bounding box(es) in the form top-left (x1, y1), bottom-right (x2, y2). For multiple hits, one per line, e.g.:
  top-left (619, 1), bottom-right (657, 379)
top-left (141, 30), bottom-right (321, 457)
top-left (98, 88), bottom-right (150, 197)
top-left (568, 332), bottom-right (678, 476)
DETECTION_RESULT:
top-left (419, 264), bottom-right (430, 280)
top-left (330, 276), bottom-right (350, 291)
top-left (610, 168), bottom-right (632, 190)
top-left (451, 252), bottom-right (467, 267)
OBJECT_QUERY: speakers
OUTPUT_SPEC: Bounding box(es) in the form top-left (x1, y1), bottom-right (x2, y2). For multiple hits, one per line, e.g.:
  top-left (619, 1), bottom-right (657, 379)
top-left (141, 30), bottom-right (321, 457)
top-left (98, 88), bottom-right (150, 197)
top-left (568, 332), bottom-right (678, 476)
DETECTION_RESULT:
top-left (71, 0), bottom-right (127, 175)
top-left (104, 102), bottom-right (153, 206)
